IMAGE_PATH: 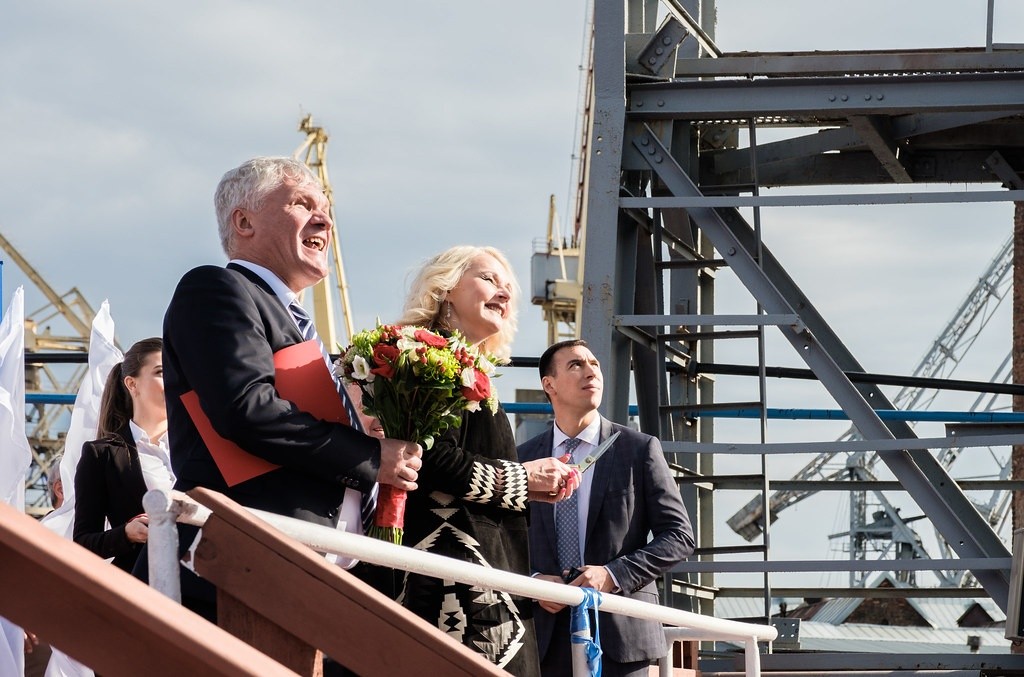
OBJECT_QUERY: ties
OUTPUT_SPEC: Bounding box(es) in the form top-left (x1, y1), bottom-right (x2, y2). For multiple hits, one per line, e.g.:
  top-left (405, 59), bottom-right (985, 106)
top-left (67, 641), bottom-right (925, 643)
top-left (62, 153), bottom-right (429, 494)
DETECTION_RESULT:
top-left (556, 437), bottom-right (582, 577)
top-left (289, 299), bottom-right (378, 537)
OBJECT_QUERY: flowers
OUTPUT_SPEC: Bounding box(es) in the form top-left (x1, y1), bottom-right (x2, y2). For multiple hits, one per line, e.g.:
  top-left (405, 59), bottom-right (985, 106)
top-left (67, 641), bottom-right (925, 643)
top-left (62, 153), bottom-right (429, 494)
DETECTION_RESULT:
top-left (333, 316), bottom-right (513, 545)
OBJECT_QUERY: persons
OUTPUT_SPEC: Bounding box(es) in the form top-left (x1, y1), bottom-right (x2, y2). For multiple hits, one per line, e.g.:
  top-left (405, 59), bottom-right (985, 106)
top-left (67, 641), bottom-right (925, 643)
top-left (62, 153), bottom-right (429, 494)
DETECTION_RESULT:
top-left (24, 450), bottom-right (64, 677)
top-left (73, 338), bottom-right (178, 677)
top-left (517, 339), bottom-right (696, 677)
top-left (393, 245), bottom-right (583, 677)
top-left (332, 358), bottom-right (385, 438)
top-left (162, 157), bottom-right (424, 677)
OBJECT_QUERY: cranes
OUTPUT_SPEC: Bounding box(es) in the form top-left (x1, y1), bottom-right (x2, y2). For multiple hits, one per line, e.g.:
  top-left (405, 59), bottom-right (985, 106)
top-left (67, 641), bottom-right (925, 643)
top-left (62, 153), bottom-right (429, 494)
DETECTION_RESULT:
top-left (526, 0), bottom-right (595, 349)
top-left (719, 229), bottom-right (1015, 590)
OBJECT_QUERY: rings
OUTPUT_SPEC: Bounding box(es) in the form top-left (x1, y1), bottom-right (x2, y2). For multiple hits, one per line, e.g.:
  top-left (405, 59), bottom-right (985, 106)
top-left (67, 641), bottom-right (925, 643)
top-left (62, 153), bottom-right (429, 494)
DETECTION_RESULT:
top-left (560, 479), bottom-right (566, 486)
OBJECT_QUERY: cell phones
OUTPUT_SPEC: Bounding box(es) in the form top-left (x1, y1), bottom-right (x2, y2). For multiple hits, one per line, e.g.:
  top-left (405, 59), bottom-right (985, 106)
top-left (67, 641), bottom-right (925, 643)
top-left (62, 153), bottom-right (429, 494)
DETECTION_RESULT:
top-left (564, 567), bottom-right (584, 585)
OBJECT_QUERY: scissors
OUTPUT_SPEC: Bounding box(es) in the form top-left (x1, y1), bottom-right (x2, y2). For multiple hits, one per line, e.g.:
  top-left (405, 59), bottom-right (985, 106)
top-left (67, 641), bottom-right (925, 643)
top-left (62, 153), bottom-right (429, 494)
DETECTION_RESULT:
top-left (560, 430), bottom-right (622, 487)
